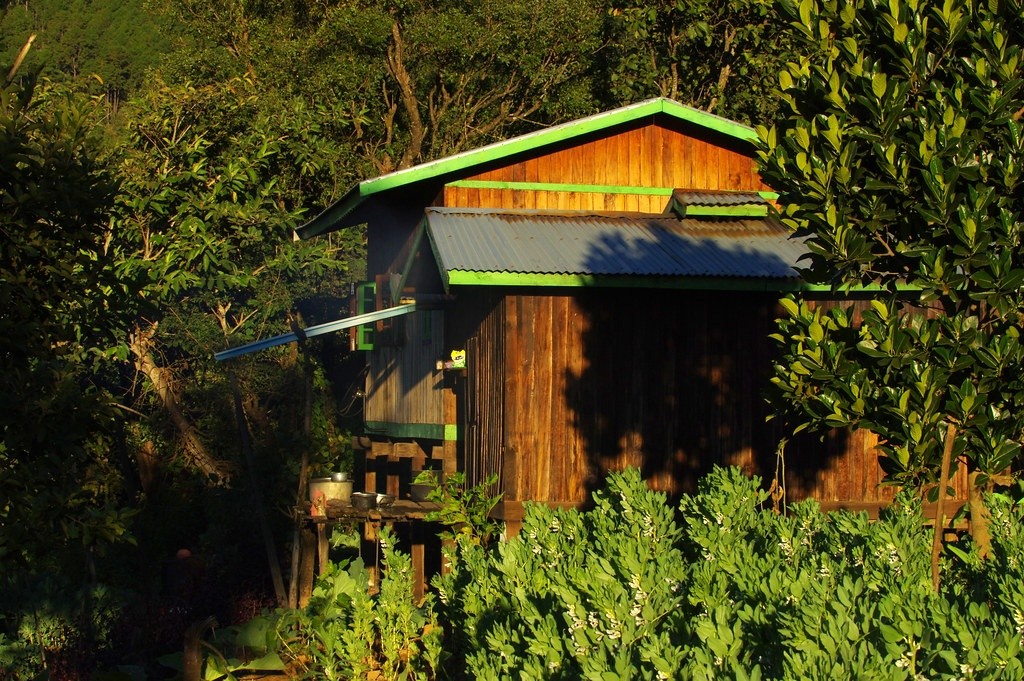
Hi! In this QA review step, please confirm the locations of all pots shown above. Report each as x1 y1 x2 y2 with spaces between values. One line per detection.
352 490 378 510
376 493 388 504
308 477 354 504
411 483 438 502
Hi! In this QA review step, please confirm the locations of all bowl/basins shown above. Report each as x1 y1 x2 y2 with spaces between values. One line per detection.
331 472 348 482
